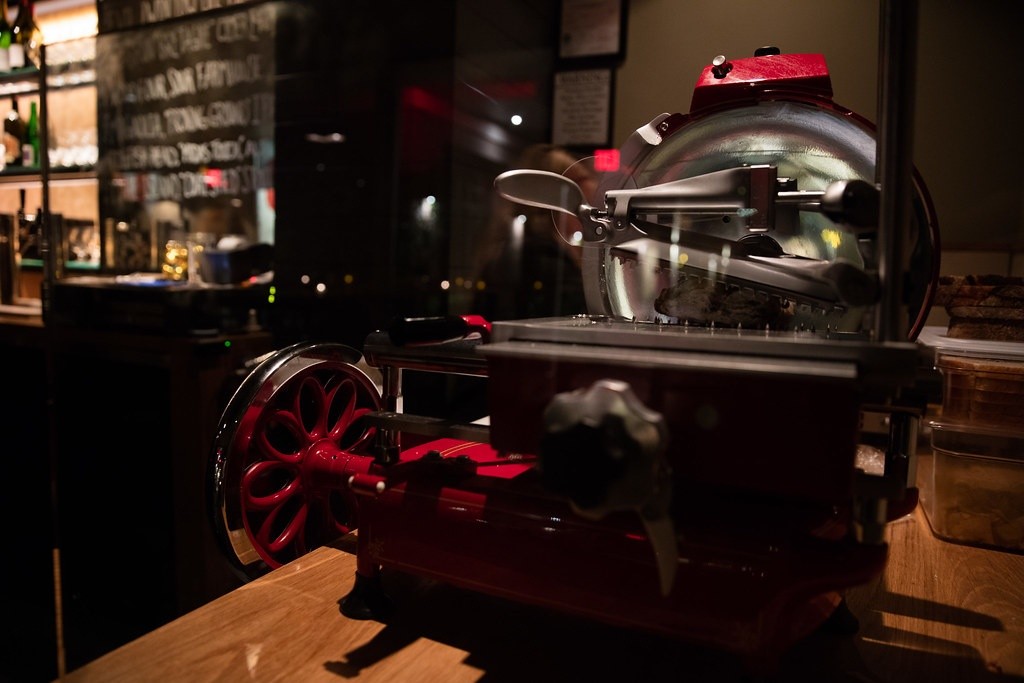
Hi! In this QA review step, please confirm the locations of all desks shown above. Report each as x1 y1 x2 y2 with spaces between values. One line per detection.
57 442 1024 683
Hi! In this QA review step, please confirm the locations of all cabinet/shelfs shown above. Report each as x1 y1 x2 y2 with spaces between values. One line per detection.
0 0 102 277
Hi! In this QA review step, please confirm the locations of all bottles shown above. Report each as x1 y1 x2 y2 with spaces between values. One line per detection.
3 96 40 168
0 0 42 74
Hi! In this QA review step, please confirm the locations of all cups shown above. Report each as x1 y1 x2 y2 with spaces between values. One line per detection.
186 232 216 282
